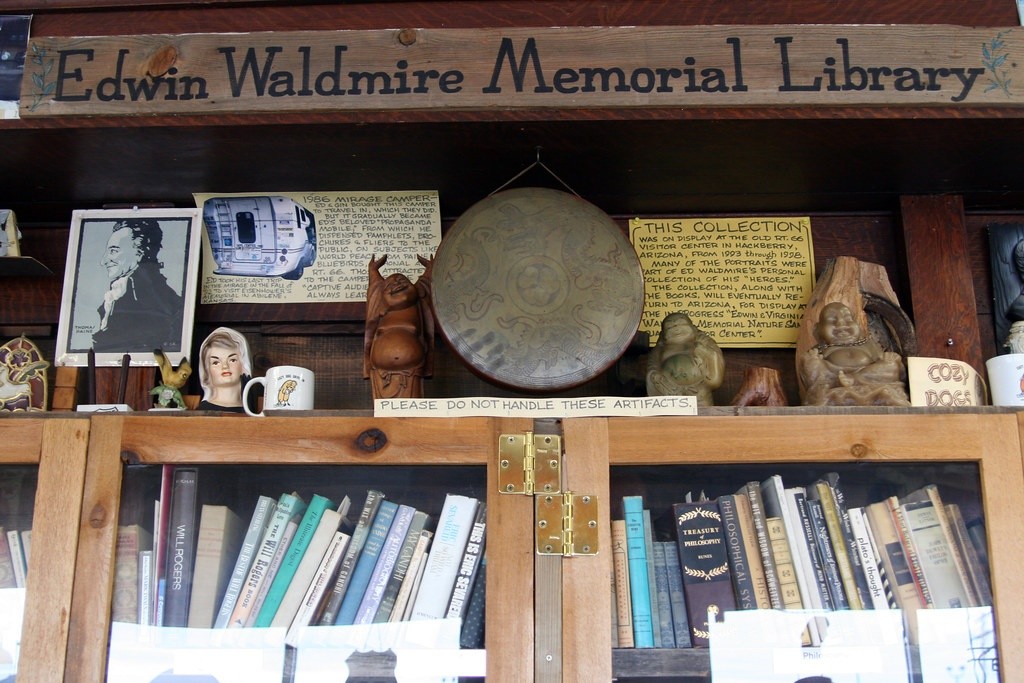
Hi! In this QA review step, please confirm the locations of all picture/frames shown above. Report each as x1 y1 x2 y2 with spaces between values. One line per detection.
55 208 205 368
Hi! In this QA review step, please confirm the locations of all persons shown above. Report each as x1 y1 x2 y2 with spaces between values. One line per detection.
366 254 435 398
646 313 724 407
199 326 257 413
801 301 909 406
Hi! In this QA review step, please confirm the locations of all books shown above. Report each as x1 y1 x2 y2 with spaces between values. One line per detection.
612 474 992 649
113 464 487 650
0 528 32 589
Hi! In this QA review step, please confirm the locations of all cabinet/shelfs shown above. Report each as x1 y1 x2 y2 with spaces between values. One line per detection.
0 406 1023 683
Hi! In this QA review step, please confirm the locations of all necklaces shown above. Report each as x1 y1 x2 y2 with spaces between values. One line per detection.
817 338 867 354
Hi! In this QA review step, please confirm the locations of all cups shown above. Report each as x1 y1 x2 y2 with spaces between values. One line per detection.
985 354 1024 406
243 366 314 417
